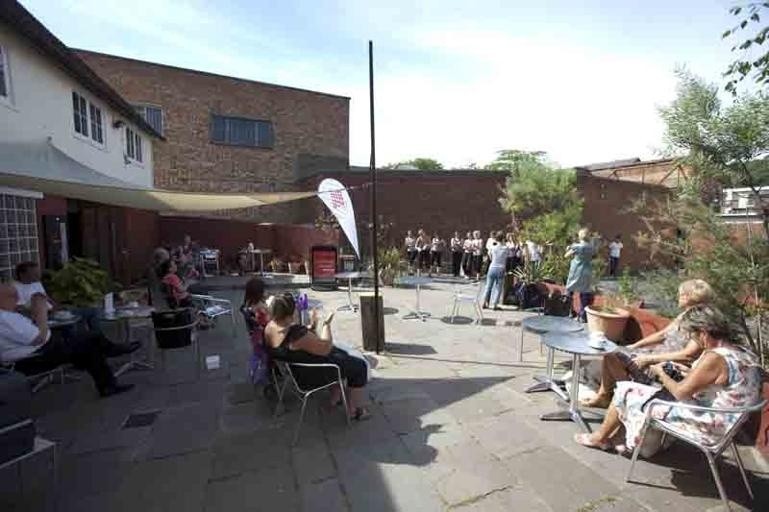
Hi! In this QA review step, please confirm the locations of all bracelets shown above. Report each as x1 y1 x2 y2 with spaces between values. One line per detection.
323 321 330 326
649 354 655 362
662 375 671 388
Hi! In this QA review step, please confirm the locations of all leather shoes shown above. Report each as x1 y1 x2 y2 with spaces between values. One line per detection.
99 385 134 397
123 341 141 354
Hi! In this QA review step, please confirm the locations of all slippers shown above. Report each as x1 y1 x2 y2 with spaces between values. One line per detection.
346 407 369 420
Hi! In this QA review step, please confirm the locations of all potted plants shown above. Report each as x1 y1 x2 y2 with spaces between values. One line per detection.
507 260 554 309
375 243 401 287
583 292 632 343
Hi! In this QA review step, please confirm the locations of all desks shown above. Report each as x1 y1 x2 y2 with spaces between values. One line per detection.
518 313 585 403
391 274 435 322
540 329 616 437
97 304 159 378
334 271 368 313
33 312 82 327
1 419 56 510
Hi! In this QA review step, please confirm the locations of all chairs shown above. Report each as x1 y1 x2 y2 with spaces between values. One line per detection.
150 246 270 362
247 326 284 402
1 359 70 393
625 393 769 512
448 282 485 325
270 358 352 447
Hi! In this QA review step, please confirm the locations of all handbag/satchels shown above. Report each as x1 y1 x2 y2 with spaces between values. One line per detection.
655 371 684 401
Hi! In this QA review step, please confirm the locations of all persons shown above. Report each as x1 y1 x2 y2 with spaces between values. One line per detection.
8 261 142 357
572 303 762 458
583 277 716 409
240 277 287 376
481 230 509 310
262 292 371 422
404 229 624 283
564 228 593 321
141 233 218 328
231 237 255 274
1 280 142 396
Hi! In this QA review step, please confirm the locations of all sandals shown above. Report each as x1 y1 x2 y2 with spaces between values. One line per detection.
581 398 610 408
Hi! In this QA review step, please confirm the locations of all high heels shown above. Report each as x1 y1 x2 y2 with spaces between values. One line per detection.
611 439 626 456
574 433 611 451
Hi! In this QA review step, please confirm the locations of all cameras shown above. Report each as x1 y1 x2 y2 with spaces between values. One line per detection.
654 362 683 383
